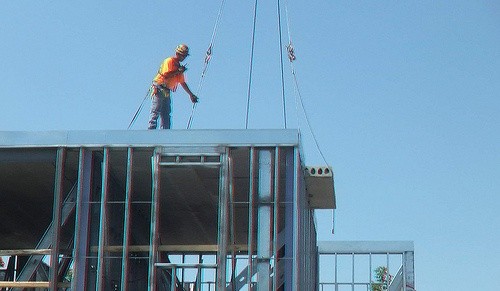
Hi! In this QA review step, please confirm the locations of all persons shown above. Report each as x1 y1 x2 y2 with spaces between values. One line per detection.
148 43 199 130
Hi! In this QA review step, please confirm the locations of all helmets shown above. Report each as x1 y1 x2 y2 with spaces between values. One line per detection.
175 44 188 57
